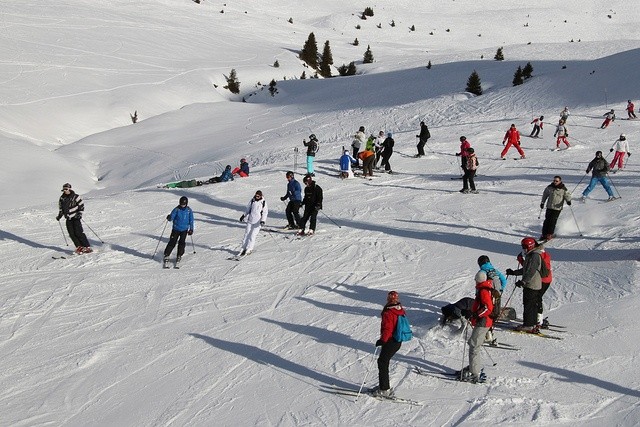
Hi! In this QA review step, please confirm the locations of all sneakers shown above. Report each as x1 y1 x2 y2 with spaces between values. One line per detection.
163 256 169 264
617 167 622 171
177 255 181 262
501 156 504 159
81 246 93 254
459 188 467 193
245 249 254 256
458 367 468 375
609 168 613 171
581 195 586 200
521 155 525 159
72 246 81 256
307 229 315 237
372 386 381 392
373 389 390 398
607 197 614 201
296 229 305 237
240 248 246 254
516 324 538 334
470 189 476 193
459 372 478 382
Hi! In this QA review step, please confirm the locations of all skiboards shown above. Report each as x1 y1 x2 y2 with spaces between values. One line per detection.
493 321 564 340
52 255 77 260
515 317 567 332
451 176 463 179
416 366 457 381
164 261 181 269
502 156 520 160
483 341 519 351
535 233 556 244
228 254 247 261
286 231 310 239
607 168 621 174
581 196 621 203
337 388 418 404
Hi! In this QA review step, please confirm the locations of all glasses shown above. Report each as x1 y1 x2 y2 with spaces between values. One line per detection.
287 176 291 178
255 195 260 198
180 203 186 206
304 183 308 185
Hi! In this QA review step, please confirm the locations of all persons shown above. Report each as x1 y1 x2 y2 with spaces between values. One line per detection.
356 150 375 177
500 123 527 163
369 290 407 397
581 151 615 204
556 104 573 130
162 191 194 271
478 255 506 342
529 116 545 138
232 158 250 178
303 133 320 177
459 147 479 193
609 132 632 172
295 173 324 237
598 109 616 129
415 121 430 157
364 130 377 153
338 150 357 179
56 183 93 255
162 178 203 189
624 99 638 121
281 170 304 231
351 124 366 164
209 165 235 184
455 135 471 177
237 189 267 262
374 130 385 170
552 120 572 152
540 175 572 241
440 273 492 383
380 131 394 173
440 296 475 337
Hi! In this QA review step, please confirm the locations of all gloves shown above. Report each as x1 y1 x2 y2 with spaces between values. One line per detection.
541 128 543 130
456 153 459 156
517 254 524 264
554 134 556 137
260 222 265 226
627 152 631 156
240 214 245 222
566 134 568 137
56 214 62 221
375 340 384 347
515 280 523 288
518 140 521 145
167 215 171 221
503 141 505 144
610 148 614 152
77 214 82 219
297 202 302 207
506 269 514 275
281 197 285 201
187 230 193 235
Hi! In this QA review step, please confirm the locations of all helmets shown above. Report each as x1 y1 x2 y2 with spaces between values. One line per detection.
286 171 294 181
511 124 515 127
303 175 312 186
521 238 536 252
387 291 399 302
559 120 564 125
478 255 490 267
226 165 231 169
309 134 315 140
180 196 188 208
565 106 569 110
467 147 474 154
240 158 246 162
459 135 466 142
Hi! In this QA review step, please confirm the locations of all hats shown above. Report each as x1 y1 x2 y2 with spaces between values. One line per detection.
61 182 72 192
475 271 487 283
256 190 262 196
344 149 349 156
619 132 626 137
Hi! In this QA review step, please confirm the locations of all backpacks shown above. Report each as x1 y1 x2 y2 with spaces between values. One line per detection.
530 252 549 278
394 314 413 343
478 286 501 319
